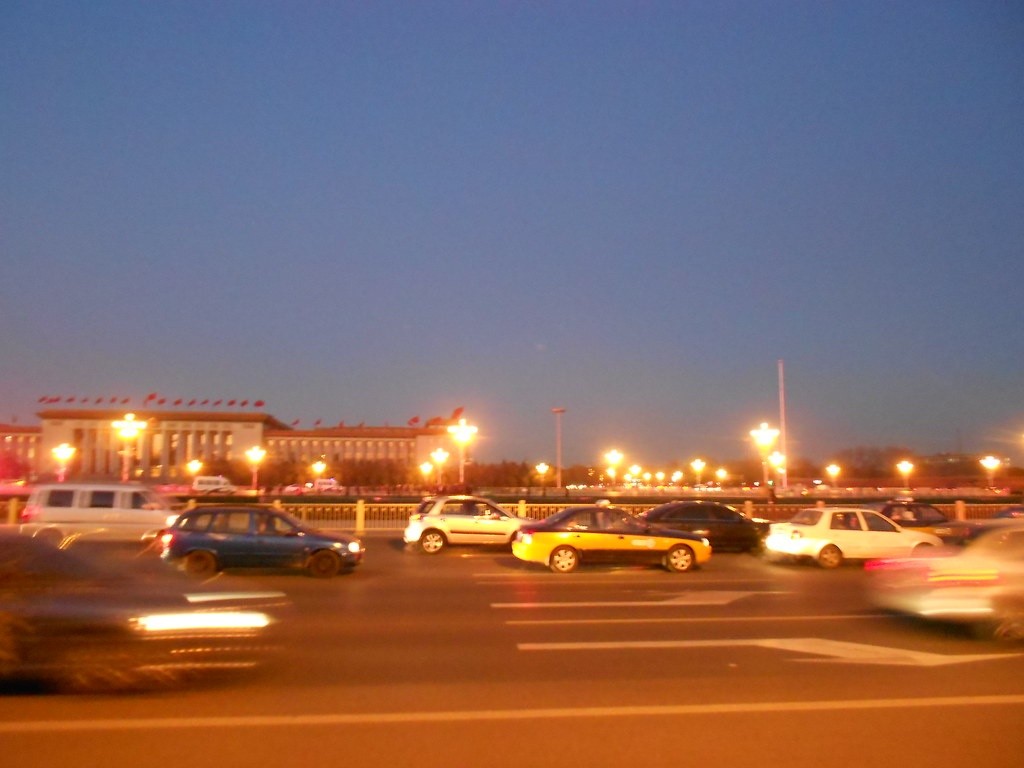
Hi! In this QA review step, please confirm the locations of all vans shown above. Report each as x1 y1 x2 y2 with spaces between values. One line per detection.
17 483 180 549
192 475 236 495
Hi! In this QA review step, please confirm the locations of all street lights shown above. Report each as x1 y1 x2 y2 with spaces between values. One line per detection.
51 444 77 480
416 418 479 486
604 418 998 493
111 414 150 482
246 445 267 491
551 407 567 487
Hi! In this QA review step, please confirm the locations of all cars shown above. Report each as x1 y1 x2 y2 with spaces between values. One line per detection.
865 529 1024 643
766 508 943 570
513 503 714 571
850 500 972 543
284 478 346 494
0 531 276 694
620 502 774 552
404 496 540 555
156 505 364 580
